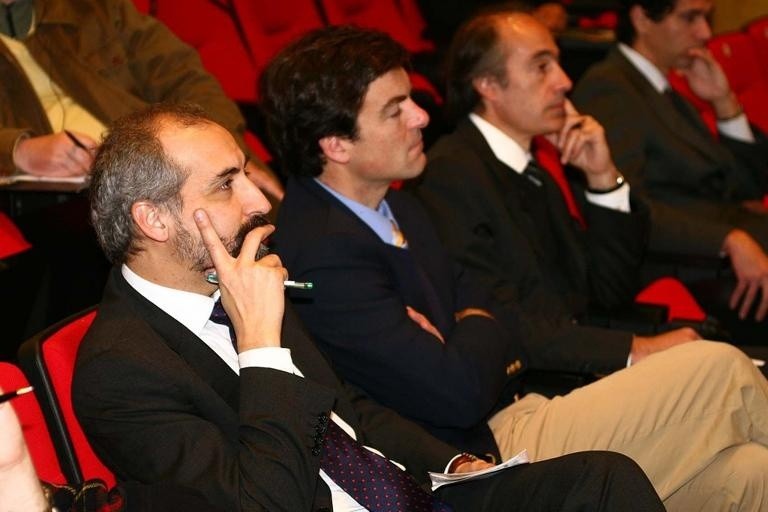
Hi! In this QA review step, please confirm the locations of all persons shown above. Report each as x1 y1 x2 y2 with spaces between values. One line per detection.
393 11 767 398
0 390 103 512
570 0 768 321
71 101 669 511
1 0 283 247
258 24 768 512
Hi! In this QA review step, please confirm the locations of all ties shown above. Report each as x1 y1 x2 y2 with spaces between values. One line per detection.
389 219 411 254
209 295 447 508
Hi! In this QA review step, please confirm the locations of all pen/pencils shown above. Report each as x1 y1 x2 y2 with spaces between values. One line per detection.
207 274 313 289
0 386 33 403
63 129 88 151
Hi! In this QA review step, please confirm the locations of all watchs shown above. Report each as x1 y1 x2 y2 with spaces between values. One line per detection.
447 454 479 474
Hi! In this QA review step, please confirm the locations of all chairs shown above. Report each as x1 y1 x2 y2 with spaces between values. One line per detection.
534 133 723 340
321 0 437 54
745 14 768 62
151 0 274 166
0 212 33 272
230 0 326 74
0 358 77 512
390 72 455 191
18 302 125 512
704 32 768 136
668 68 768 215
